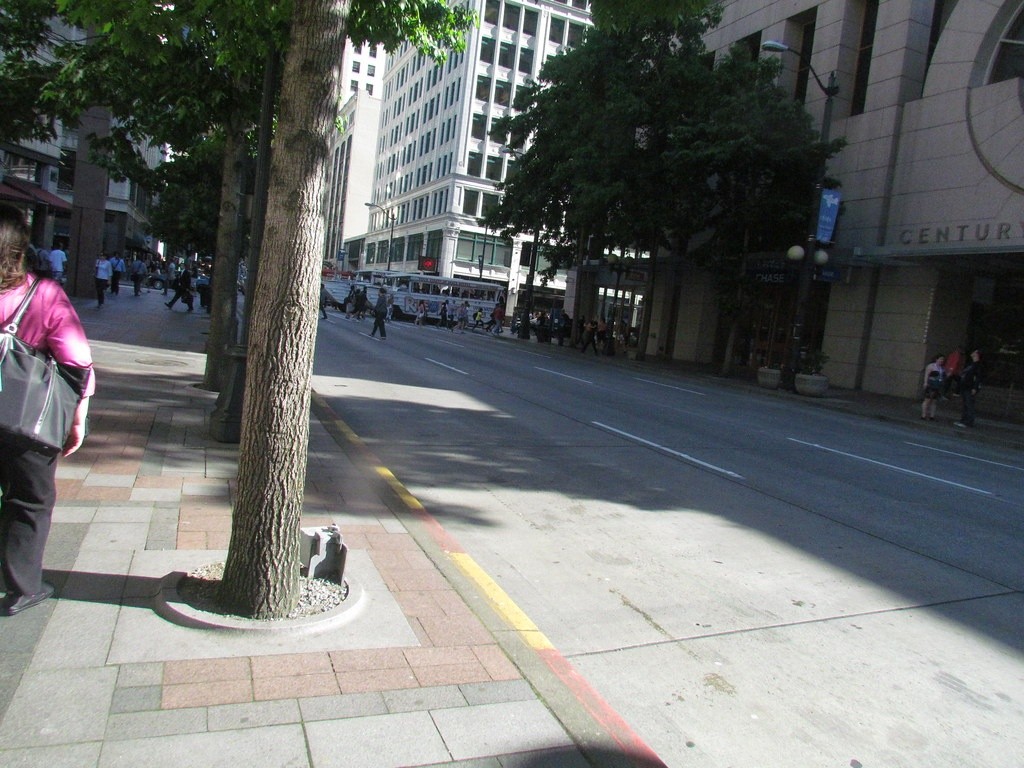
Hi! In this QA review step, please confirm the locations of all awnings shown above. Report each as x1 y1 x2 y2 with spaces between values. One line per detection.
126 238 158 256
0 176 72 210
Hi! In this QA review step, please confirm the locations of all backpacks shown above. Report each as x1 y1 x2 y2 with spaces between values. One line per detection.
966 374 984 405
927 370 943 391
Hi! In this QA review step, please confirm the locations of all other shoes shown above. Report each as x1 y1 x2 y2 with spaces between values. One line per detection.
2 580 55 614
164 303 172 308
187 308 193 311
161 292 166 295
921 415 939 422
953 420 967 428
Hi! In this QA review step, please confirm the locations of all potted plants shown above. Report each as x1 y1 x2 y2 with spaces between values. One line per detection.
795 348 832 397
757 355 781 388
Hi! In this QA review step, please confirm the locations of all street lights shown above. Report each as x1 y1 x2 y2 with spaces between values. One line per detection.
762 39 837 361
502 149 539 338
365 203 396 272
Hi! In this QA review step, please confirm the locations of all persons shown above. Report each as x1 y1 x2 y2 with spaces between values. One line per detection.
320 283 329 320
415 283 630 357
344 284 394 341
27 240 213 313
0 202 96 613
920 345 987 428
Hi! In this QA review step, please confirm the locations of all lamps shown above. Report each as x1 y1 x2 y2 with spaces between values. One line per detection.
761 41 844 97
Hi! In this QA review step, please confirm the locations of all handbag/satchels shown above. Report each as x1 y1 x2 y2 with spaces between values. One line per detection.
473 313 478 320
90 258 101 278
130 272 134 281
376 303 388 319
1 273 93 456
181 291 194 303
418 312 425 318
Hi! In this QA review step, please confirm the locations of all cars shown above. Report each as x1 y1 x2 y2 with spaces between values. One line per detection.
146 271 209 294
